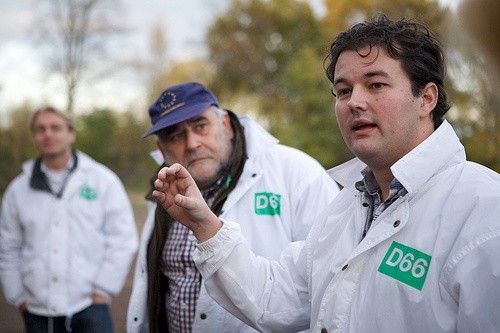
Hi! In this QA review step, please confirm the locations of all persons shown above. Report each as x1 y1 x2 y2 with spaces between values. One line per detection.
152 14 500 332
1 106 139 332
125 82 340 333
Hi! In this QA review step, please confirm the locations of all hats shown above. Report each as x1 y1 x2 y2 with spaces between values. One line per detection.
142 82 219 141
